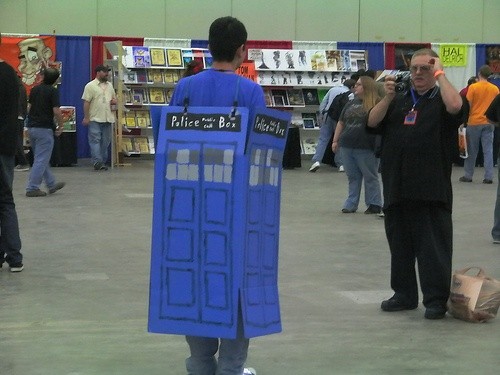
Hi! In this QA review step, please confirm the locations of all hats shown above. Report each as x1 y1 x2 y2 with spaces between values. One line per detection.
95 65 111 72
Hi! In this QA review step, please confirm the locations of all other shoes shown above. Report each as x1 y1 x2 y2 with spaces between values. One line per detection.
460 176 472 182
492 239 500 244
49 182 65 194
26 189 46 197
338 165 345 172
483 179 493 184
94 164 108 171
364 204 381 215
341 208 356 213
243 367 256 375
308 161 320 172
381 295 418 311
425 307 446 319
9 264 24 272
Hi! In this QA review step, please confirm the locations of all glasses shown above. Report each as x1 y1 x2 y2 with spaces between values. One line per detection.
354 83 362 86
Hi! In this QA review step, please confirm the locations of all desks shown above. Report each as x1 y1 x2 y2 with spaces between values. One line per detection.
28 131 78 167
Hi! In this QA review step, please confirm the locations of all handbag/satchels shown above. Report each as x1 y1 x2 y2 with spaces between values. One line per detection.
448 265 500 323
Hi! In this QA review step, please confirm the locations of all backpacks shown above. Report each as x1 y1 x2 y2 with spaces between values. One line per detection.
322 91 353 121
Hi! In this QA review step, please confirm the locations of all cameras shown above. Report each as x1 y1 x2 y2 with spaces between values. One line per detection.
393 74 411 93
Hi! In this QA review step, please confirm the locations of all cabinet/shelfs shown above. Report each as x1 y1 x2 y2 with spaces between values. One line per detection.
104 41 369 167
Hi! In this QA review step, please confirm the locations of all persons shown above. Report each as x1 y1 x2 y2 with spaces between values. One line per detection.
365 49 470 320
332 76 386 214
309 79 356 172
81 66 117 171
484 93 500 244
183 60 203 77
461 64 499 184
459 76 479 96
0 58 27 272
26 68 66 197
169 16 266 375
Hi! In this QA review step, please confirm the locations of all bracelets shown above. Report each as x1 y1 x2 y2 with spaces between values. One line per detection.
434 70 445 80
